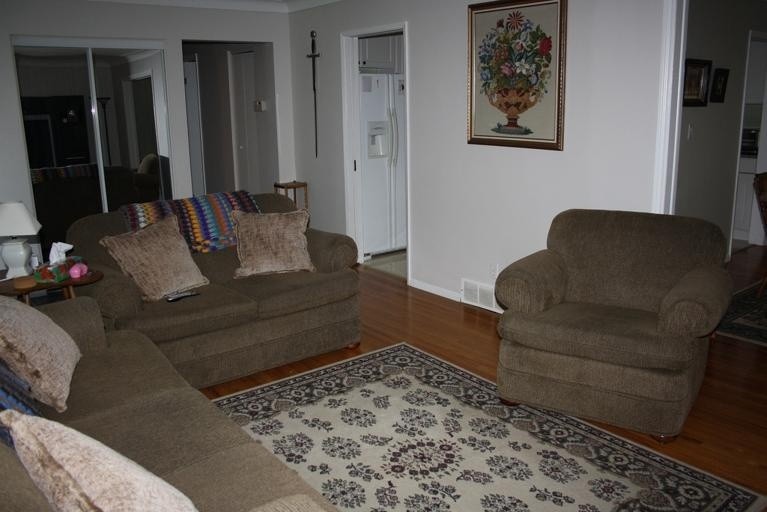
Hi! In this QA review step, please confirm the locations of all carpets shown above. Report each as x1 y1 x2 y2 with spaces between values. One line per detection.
211 340 767 512
713 278 767 347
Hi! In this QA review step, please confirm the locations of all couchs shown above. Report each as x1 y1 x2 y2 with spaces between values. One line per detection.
33 150 171 263
0 295 341 512
64 193 364 391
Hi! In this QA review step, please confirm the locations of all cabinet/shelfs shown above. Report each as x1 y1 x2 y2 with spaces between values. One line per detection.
357 33 405 75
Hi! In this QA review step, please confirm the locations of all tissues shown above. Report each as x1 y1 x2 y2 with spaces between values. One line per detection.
34 241 85 284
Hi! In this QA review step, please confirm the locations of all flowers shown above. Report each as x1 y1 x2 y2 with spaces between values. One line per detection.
478 10 553 102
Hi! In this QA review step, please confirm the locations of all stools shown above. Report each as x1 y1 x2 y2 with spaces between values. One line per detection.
274 179 310 229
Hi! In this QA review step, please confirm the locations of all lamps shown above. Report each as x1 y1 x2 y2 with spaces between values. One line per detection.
0 201 43 280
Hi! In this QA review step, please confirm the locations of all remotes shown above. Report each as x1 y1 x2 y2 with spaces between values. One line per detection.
165 289 202 302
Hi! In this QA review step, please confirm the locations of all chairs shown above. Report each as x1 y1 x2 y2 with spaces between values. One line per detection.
495 208 735 444
752 172 767 299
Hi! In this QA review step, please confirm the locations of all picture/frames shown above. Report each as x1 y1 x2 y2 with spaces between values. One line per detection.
709 67 731 104
466 0 568 149
682 58 713 107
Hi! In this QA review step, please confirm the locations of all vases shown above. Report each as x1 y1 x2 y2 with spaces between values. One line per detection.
487 87 542 135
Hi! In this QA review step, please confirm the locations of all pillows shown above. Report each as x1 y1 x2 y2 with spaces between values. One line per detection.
0 408 199 512
0 294 85 414
231 206 317 281
98 214 210 303
